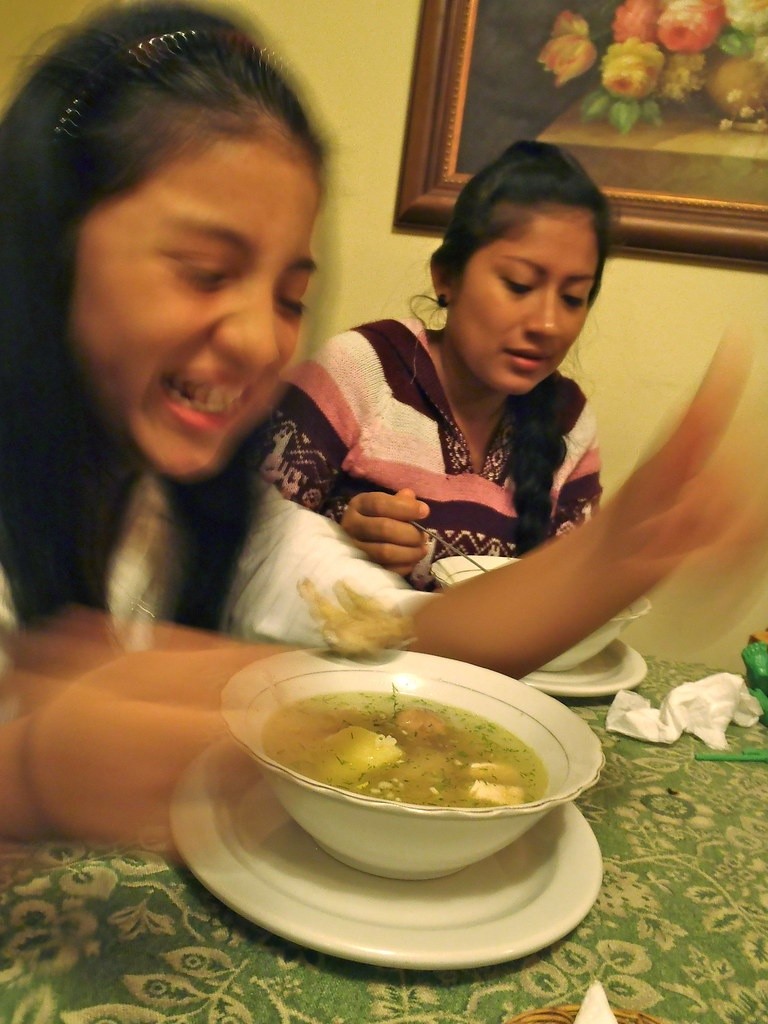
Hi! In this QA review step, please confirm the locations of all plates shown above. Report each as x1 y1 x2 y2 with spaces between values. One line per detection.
514 637 647 697
171 734 603 971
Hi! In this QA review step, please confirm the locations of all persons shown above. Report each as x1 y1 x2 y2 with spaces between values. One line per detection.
257 139 612 609
1 4 755 871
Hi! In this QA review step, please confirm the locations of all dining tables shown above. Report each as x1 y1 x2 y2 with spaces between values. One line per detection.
2 649 768 1024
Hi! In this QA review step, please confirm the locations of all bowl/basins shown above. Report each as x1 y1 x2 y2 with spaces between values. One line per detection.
429 555 651 674
217 647 609 889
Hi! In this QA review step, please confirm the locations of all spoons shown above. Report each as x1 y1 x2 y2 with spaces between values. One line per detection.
130 597 415 664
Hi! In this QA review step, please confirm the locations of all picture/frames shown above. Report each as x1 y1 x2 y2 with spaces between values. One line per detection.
391 1 767 268
